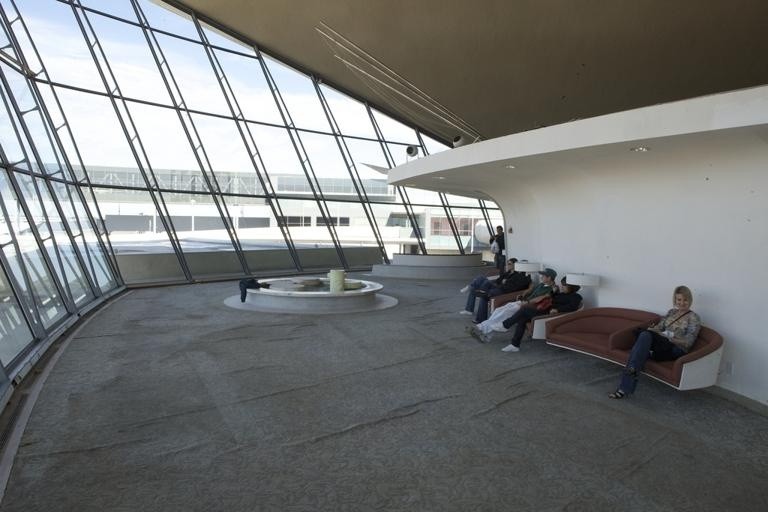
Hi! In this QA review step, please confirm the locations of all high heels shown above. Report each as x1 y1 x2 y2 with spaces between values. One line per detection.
607 389 631 399
621 366 640 379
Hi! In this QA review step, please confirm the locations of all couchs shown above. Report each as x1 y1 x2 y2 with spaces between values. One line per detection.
472 274 723 393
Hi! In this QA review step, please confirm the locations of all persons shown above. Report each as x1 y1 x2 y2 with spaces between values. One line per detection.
457 257 516 314
462 268 559 344
492 276 582 353
471 271 530 323
607 285 701 399
490 225 507 274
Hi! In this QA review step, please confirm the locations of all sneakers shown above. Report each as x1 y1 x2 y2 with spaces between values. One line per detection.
490 321 509 332
501 344 520 352
460 285 473 294
460 309 491 344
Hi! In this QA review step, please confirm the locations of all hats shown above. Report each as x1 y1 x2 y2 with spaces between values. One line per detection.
538 268 557 278
